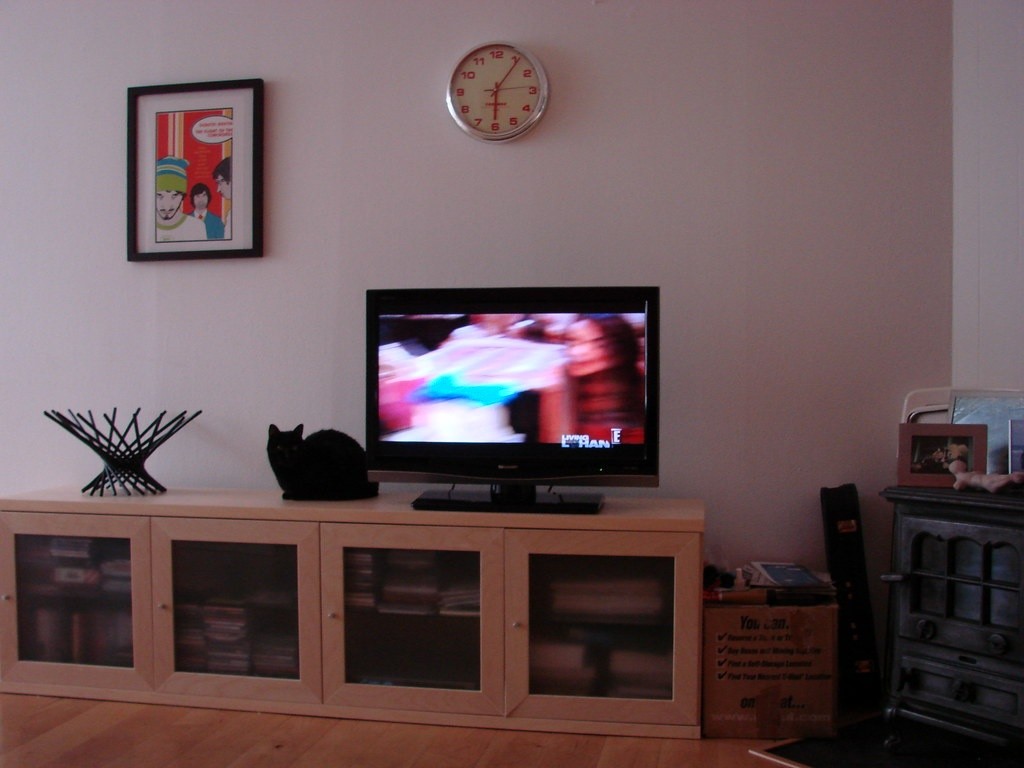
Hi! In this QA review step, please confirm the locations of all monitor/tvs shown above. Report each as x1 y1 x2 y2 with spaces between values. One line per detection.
366 285 662 515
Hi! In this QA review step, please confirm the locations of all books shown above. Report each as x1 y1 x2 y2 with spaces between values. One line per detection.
176 602 298 676
743 561 837 595
345 551 480 617
20 537 132 659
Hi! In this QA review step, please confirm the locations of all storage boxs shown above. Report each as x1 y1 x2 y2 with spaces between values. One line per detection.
878 486 1024 756
703 594 838 738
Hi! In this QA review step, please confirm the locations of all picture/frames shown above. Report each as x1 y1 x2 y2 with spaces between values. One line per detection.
896 423 988 485
125 78 263 260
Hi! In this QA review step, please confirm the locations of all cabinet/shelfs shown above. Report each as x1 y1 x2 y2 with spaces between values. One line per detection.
0 485 704 740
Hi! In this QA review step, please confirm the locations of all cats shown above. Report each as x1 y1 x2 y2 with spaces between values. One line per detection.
266 424 379 501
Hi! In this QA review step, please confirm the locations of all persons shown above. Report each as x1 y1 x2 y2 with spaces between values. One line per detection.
911 444 968 473
380 312 645 444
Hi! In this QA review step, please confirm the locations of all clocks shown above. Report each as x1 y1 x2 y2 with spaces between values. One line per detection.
445 39 550 142
